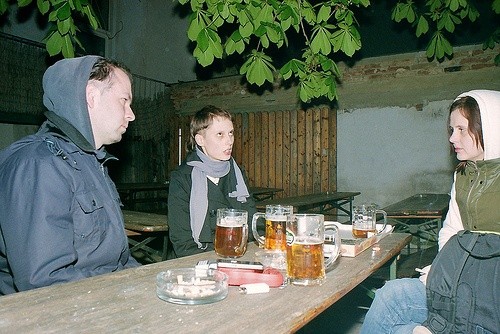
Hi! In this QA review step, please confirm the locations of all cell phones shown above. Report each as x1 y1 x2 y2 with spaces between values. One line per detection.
217 258 263 270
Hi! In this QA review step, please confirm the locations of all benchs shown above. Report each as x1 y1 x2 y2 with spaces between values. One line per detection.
343 212 384 227
124 228 158 263
389 218 412 255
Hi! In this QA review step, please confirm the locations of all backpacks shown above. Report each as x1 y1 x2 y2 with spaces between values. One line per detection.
422 228 500 334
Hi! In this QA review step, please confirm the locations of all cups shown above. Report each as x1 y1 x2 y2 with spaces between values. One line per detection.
286 214 342 287
214 208 248 259
251 205 293 253
352 205 387 238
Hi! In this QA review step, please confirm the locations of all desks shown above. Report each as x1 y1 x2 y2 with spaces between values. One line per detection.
0 233 413 334
121 210 169 263
252 186 285 201
377 192 451 253
256 190 361 221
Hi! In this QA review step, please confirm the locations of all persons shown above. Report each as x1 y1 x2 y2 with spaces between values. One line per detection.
0 54 144 296
169 105 266 259
360 89 500 334
